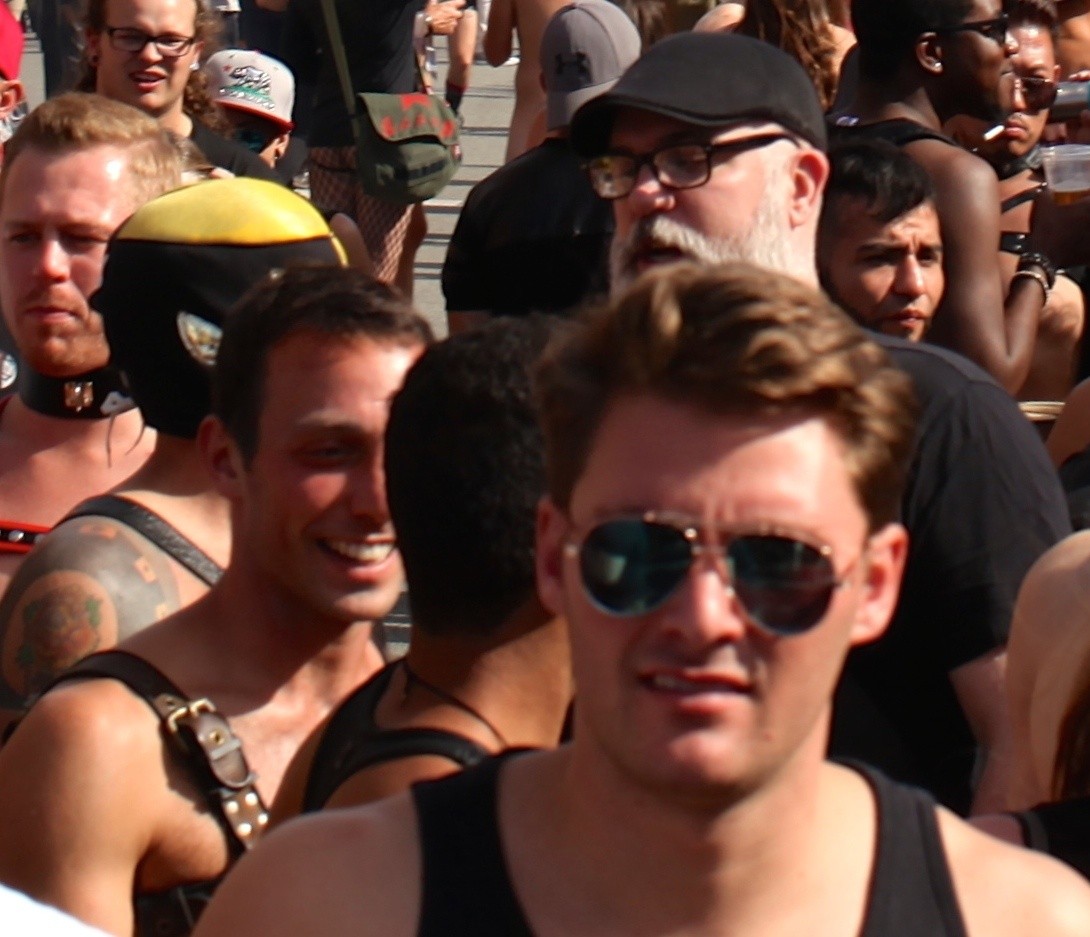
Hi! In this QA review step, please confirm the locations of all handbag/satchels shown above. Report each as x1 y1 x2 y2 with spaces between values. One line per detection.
348 92 462 205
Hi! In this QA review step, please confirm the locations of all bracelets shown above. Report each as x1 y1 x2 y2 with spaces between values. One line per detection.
1009 270 1049 309
1013 251 1056 289
422 10 433 38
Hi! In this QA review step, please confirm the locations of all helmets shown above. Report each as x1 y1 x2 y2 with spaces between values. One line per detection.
103 178 348 438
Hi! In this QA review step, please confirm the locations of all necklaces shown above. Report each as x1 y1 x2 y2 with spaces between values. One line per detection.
398 659 514 755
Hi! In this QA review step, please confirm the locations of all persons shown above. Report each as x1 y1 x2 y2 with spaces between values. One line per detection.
0 0 1090 937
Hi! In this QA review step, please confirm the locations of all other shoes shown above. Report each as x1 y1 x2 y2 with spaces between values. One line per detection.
423 46 441 77
474 51 521 66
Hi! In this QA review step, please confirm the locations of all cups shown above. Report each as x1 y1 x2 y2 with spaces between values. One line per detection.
1038 144 1090 205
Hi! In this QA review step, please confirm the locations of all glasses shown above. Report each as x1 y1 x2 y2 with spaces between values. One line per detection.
1013 76 1065 113
579 130 801 199
214 130 283 155
97 20 197 57
558 511 866 638
933 10 1010 45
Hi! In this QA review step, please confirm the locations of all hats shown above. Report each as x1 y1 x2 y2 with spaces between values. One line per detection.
0 1 24 81
203 49 295 131
567 32 826 156
539 0 641 131
